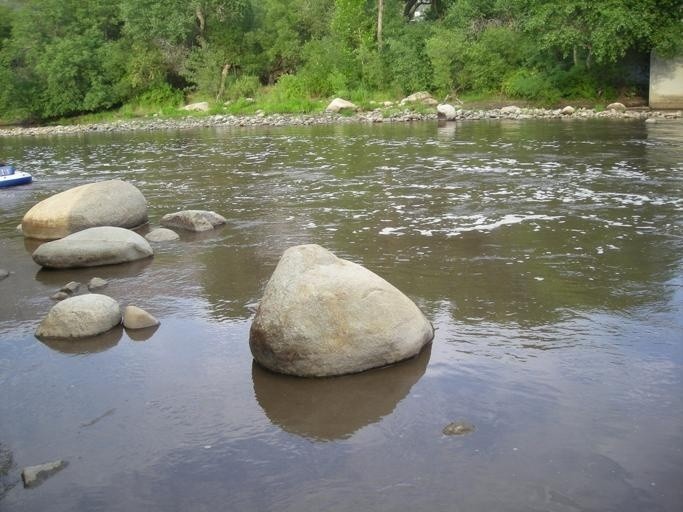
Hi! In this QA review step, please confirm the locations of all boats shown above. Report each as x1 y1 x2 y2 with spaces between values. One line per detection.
0 164 33 187
